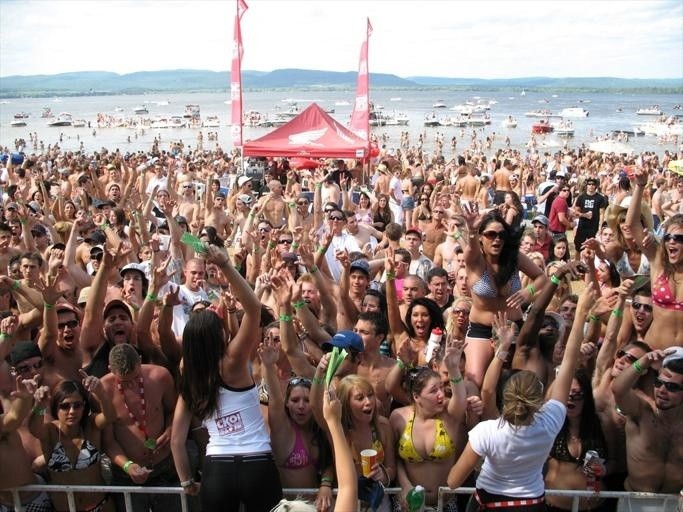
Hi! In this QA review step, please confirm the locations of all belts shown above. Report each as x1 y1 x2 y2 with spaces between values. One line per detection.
204 453 277 462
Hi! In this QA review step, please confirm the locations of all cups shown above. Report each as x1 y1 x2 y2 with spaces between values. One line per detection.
360 449 377 477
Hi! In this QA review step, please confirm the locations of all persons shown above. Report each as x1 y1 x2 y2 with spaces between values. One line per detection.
0 98 682 511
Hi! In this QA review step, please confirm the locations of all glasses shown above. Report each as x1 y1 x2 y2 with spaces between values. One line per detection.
0 178 683 412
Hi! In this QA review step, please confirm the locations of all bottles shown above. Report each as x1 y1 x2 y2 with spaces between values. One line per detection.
406 485 425 511
425 327 442 363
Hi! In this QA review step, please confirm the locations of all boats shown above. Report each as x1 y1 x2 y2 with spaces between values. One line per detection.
502 102 681 137
